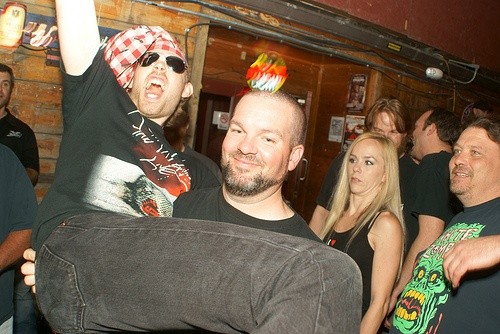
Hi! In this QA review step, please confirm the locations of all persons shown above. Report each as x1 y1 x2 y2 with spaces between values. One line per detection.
0 143 37 334
0 63 40 187
308 94 500 334
21 89 323 293
30 1 362 334
163 103 222 187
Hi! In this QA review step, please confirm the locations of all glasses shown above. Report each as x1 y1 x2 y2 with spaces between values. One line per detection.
139 51 188 74
